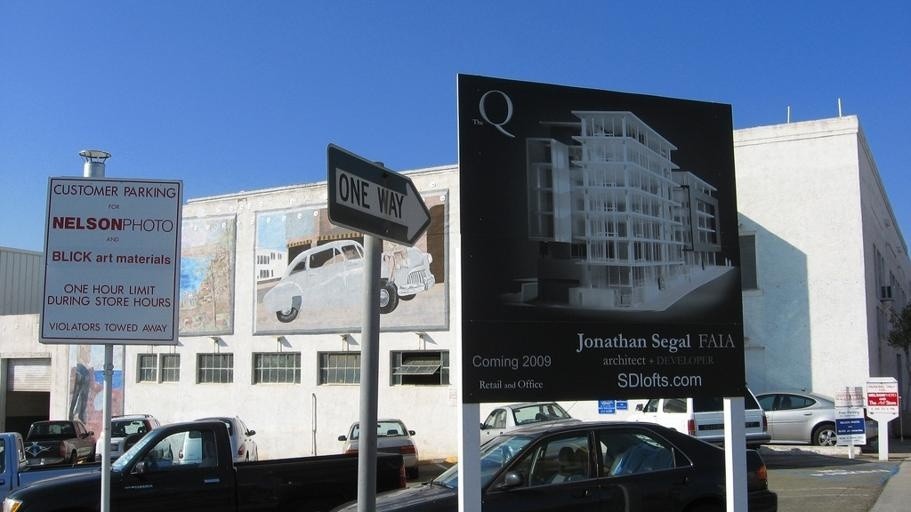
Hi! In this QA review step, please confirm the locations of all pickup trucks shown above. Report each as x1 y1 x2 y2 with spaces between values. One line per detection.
1 419 406 511
0 420 96 512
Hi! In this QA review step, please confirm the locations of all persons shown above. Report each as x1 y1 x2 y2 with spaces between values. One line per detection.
69 345 92 425
381 240 398 288
394 244 411 270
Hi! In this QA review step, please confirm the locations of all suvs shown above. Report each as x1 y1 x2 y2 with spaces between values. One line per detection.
93 413 174 464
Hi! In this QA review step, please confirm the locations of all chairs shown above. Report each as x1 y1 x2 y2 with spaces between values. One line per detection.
548 446 588 483
198 440 217 466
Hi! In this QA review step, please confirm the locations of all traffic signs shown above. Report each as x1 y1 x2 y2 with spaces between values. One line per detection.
325 141 433 249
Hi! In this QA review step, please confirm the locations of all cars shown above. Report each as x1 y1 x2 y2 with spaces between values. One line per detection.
178 415 259 466
336 418 420 480
332 418 779 512
